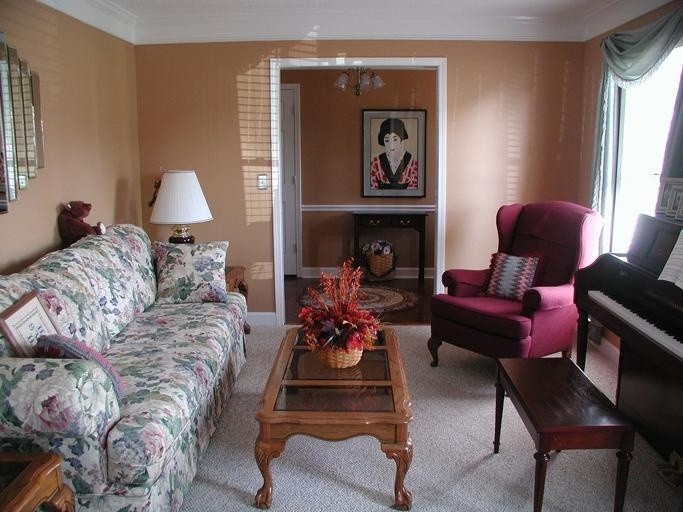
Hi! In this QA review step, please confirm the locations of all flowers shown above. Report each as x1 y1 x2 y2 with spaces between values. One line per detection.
359 239 399 270
296 255 383 352
289 380 377 419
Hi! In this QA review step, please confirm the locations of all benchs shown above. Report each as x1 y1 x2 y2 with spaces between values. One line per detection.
491 355 636 512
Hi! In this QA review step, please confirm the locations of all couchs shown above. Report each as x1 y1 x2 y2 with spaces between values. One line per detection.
0 222 248 512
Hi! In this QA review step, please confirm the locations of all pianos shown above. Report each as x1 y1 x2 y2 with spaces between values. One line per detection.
576 212 683 469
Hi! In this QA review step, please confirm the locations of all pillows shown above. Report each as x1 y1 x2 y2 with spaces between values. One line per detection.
151 239 230 305
32 333 124 402
479 250 546 304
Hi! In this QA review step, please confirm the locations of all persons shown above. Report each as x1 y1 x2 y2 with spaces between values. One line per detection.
371 118 417 190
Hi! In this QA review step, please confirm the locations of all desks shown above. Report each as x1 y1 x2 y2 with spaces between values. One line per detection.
225 265 251 334
351 211 429 286
0 451 77 512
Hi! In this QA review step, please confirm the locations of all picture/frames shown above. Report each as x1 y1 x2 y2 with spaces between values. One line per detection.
361 108 426 199
654 176 682 222
0 288 62 358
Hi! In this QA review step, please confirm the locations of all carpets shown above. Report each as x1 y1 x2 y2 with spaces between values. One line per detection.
298 280 419 315
174 324 681 511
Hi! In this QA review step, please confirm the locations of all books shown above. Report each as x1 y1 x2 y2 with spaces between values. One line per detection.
658 228 683 291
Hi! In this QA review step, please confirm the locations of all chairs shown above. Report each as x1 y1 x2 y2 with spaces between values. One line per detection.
426 201 603 396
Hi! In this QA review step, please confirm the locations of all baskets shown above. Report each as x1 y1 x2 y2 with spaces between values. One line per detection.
316 347 363 369
367 254 393 278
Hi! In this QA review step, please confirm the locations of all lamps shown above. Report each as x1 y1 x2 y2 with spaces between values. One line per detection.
147 168 214 243
332 69 386 96
